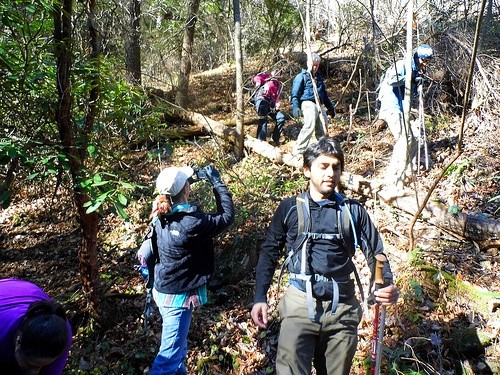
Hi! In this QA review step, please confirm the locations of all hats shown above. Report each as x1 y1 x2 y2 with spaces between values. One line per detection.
156 166 194 195
416 43 434 58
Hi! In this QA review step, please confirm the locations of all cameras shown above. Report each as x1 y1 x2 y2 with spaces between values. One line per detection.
196 170 208 179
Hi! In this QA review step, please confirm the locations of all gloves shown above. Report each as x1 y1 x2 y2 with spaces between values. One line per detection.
326 108 336 117
293 107 302 119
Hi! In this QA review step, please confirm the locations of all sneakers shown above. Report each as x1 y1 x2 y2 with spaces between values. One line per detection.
273 133 286 141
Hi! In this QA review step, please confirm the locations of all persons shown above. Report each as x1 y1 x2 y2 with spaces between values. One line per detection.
251 140 398 373
292 53 335 155
254 72 285 145
377 44 433 186
0 278 72 374
147 166 235 374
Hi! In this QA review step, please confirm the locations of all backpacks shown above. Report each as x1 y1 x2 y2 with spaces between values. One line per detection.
137 237 156 314
